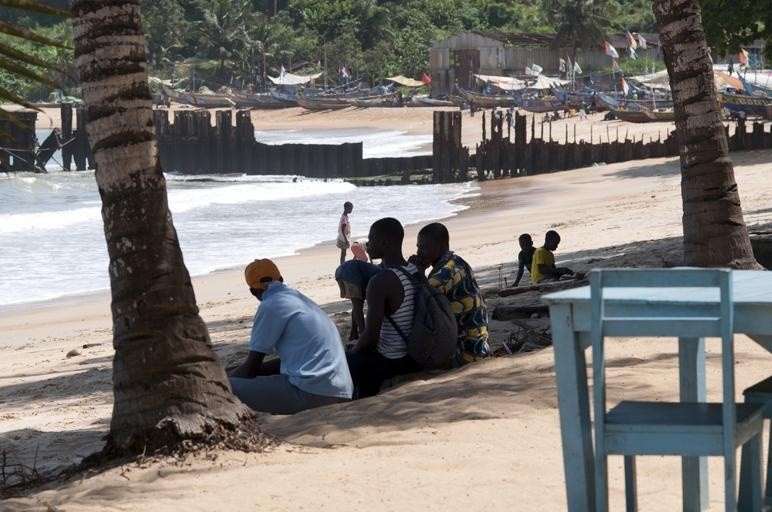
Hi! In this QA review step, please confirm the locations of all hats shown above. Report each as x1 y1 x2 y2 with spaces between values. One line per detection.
243 258 282 290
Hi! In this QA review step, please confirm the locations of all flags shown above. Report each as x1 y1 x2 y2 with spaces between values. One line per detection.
423 72 430 84
340 66 351 79
727 48 760 76
524 30 648 98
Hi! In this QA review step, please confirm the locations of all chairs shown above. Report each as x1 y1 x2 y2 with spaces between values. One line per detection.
739 374 772 511
585 268 764 510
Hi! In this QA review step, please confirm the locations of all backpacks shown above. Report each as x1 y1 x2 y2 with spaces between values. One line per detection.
385 262 458 368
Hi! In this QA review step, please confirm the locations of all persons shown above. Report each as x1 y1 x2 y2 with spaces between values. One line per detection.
511 233 536 287
350 241 373 264
408 222 491 364
377 85 600 129
226 258 355 416
346 217 426 399
335 260 382 341
530 230 573 285
335 201 353 264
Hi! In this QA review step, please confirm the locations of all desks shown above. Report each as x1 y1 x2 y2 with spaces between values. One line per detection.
540 271 772 510
